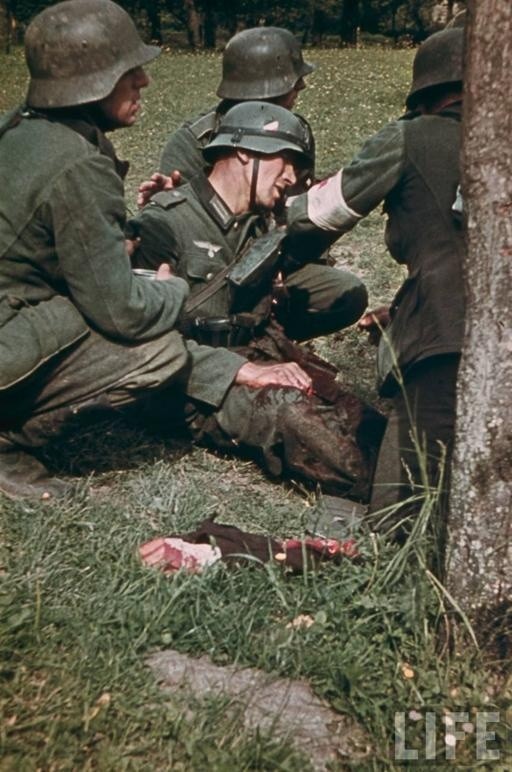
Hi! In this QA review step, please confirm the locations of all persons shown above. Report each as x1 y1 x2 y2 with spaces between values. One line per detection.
0 0 190 504
137 27 369 343
123 99 389 502
134 522 369 572
286 24 465 543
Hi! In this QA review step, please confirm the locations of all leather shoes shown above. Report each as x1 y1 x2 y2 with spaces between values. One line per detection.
0 445 88 514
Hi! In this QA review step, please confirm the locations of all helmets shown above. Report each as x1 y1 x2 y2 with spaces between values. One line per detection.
216 26 315 101
23 0 162 110
404 27 465 111
201 100 316 166
